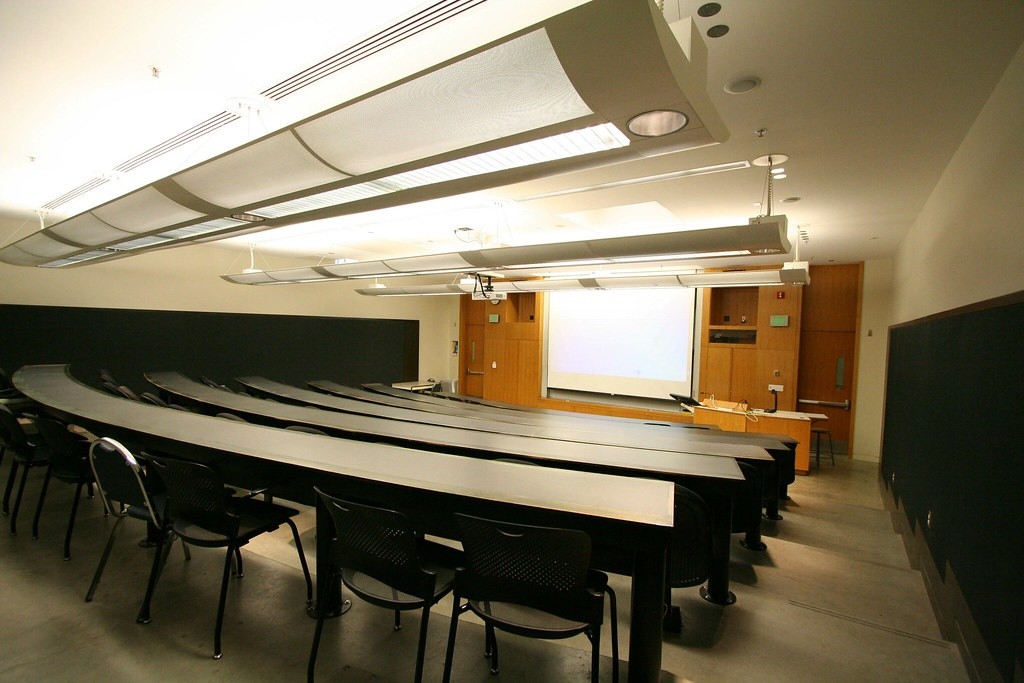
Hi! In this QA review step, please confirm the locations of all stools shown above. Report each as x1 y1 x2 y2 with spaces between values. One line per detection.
811 428 835 467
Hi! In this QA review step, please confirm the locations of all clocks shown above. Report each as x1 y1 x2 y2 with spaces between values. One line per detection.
490 299 500 305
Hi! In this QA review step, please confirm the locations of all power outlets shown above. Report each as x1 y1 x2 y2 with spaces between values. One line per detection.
773 370 779 375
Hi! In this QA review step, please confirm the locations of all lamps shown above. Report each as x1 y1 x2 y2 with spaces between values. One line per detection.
0 0 813 299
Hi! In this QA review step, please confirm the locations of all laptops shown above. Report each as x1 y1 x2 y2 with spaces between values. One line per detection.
670 393 702 407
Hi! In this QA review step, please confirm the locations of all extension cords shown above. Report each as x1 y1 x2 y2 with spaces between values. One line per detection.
718 407 733 412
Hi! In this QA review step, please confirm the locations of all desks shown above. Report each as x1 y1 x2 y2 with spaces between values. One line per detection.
11 365 830 683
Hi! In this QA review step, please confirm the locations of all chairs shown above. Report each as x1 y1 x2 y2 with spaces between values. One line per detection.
0 403 94 537
84 438 237 624
31 414 110 561
305 486 492 683
662 482 715 634
443 511 619 683
420 383 442 397
135 449 315 660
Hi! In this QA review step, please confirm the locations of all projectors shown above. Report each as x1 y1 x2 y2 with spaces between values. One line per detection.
472 291 507 300
